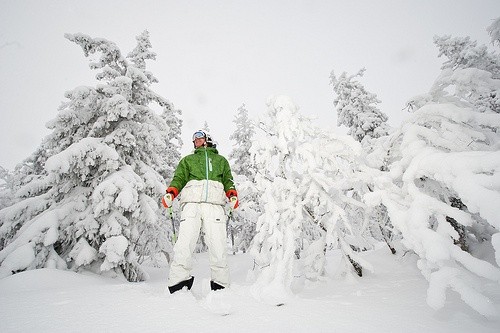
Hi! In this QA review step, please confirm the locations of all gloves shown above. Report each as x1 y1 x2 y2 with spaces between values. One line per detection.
226 190 240 209
162 187 179 208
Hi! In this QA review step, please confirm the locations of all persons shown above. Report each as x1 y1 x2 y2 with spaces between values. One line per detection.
161 129 239 294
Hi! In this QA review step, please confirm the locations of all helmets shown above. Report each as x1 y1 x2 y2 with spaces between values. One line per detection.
192 129 213 149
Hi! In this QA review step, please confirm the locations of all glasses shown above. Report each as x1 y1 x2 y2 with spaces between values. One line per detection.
192 132 205 141
